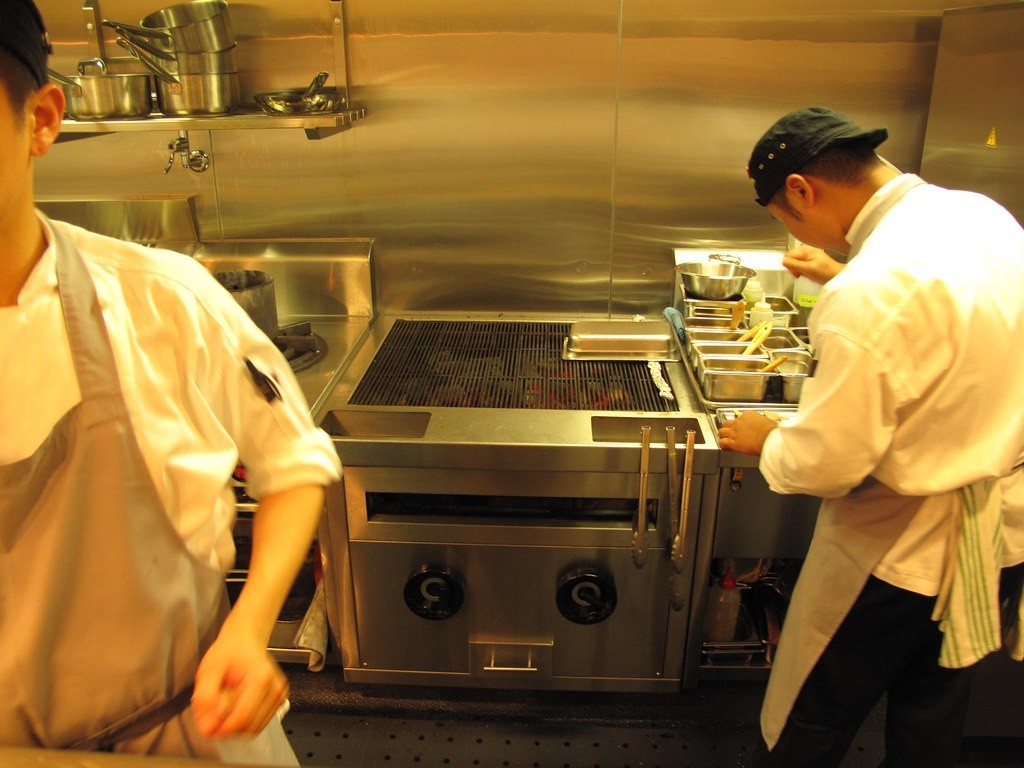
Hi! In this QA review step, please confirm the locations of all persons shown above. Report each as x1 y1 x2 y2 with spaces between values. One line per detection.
718 106 1024 768
0 0 343 768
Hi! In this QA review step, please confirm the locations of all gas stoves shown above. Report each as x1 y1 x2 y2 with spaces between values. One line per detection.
191 238 381 425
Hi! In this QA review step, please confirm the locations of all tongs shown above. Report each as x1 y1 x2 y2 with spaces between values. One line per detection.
631 424 697 576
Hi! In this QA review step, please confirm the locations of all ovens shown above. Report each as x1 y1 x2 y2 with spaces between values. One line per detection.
328 464 703 711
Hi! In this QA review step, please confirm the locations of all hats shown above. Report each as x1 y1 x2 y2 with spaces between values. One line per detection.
0 0 53 88
746 106 888 207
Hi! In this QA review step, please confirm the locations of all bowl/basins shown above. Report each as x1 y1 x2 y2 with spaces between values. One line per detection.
676 261 757 301
682 295 814 408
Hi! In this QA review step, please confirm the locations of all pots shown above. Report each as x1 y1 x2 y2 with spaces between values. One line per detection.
252 70 346 116
46 0 243 118
210 269 280 343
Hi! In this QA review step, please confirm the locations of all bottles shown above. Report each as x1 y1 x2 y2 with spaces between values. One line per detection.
703 566 741 642
